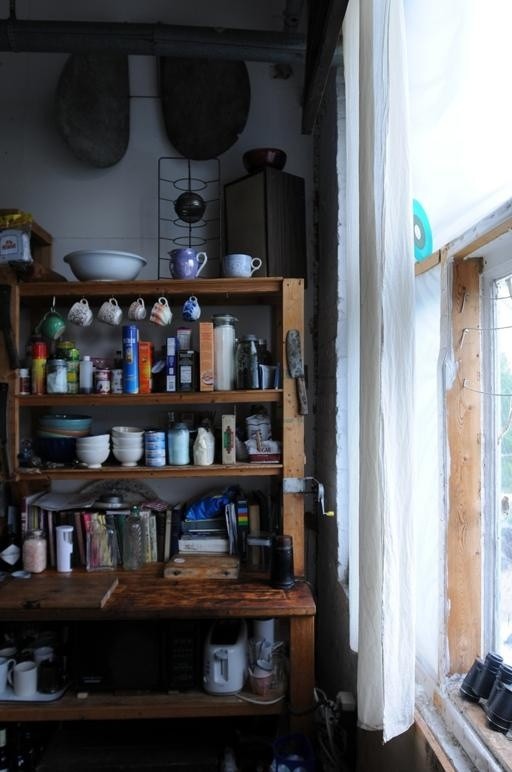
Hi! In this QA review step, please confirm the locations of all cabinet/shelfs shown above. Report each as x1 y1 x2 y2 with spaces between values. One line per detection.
0 276 306 577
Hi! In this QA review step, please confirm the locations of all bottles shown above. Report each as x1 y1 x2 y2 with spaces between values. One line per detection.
211 312 274 390
221 749 238 772
268 535 297 590
169 423 191 466
15 325 142 396
2 505 146 574
0 721 38 772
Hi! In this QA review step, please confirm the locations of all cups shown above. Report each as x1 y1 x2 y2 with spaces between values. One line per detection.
65 299 96 327
43 311 65 343
142 431 167 466
128 298 147 322
183 297 202 323
149 298 174 328
98 295 124 326
0 647 62 695
168 249 208 281
247 616 277 694
221 253 264 279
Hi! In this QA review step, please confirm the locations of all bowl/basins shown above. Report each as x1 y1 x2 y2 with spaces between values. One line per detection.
112 426 145 466
60 249 147 281
37 414 93 438
75 433 110 468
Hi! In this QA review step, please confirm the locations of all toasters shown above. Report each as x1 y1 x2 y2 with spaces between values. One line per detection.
199 618 248 693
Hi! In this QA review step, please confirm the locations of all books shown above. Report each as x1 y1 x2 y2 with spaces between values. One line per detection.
20 490 278 581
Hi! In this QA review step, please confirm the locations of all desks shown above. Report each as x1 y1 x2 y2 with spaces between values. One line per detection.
0 560 318 772
